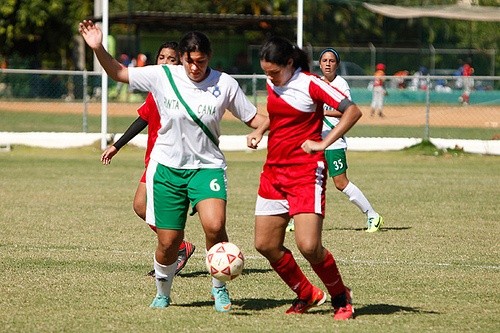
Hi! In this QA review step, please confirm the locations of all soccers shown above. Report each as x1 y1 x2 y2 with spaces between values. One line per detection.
206 242 244 282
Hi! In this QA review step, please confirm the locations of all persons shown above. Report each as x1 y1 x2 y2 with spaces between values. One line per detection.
280 49 383 234
100 41 196 279
78 18 269 313
121 47 475 121
246 39 363 319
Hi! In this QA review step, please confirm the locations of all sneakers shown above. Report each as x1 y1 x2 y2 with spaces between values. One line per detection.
286 286 327 313
150 294 172 307
331 286 355 320
174 240 196 275
284 219 295 231
211 283 231 311
365 213 383 232
